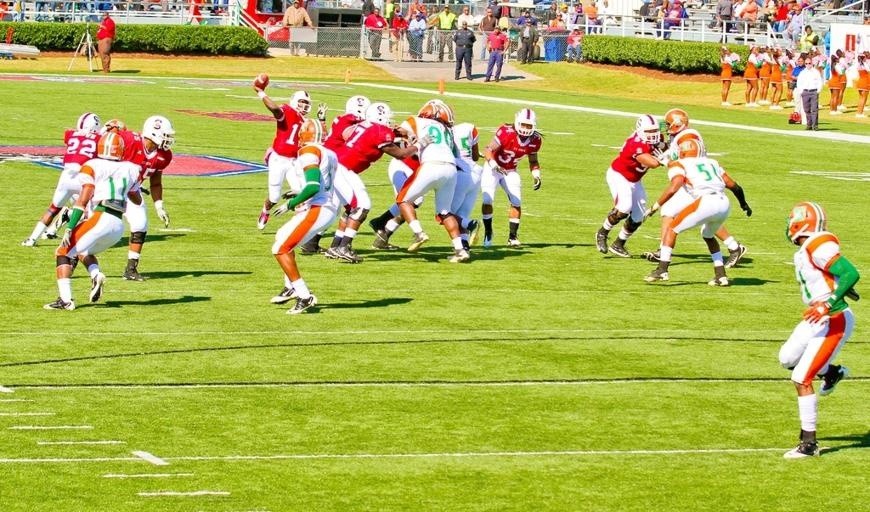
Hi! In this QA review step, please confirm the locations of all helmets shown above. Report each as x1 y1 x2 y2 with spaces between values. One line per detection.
514 108 536 136
345 95 393 126
79 112 175 159
289 91 312 115
419 99 456 124
785 201 826 245
297 118 325 147
638 109 707 154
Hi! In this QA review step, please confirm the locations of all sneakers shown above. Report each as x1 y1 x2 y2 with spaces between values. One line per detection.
720 100 864 120
44 298 76 312
304 221 522 261
88 272 106 303
818 364 848 396
122 267 144 282
594 234 745 285
257 208 270 230
270 288 319 312
783 442 819 459
24 210 66 246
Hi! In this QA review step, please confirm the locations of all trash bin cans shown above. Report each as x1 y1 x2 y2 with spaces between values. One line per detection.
542 29 570 62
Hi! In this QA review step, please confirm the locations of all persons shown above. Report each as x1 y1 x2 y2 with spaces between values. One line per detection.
96 12 116 73
268 119 339 315
361 0 869 131
641 108 749 268
21 111 174 312
642 133 754 290
778 200 862 463
254 73 544 265
594 114 672 259
283 0 315 57
0 0 283 25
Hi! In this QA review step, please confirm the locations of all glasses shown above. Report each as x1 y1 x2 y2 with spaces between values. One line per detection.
805 62 811 65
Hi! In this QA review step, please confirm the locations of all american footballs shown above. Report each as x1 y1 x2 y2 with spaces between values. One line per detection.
255 74 269 91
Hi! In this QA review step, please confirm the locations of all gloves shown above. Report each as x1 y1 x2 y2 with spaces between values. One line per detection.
532 176 541 191
741 203 752 217
60 228 73 245
154 200 170 229
803 301 829 324
316 102 328 121
413 134 435 152
643 202 660 218
491 164 507 179
652 148 670 167
272 199 292 217
280 189 299 200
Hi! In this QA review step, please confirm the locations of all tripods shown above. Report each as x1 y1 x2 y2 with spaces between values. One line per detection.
68 22 100 73
